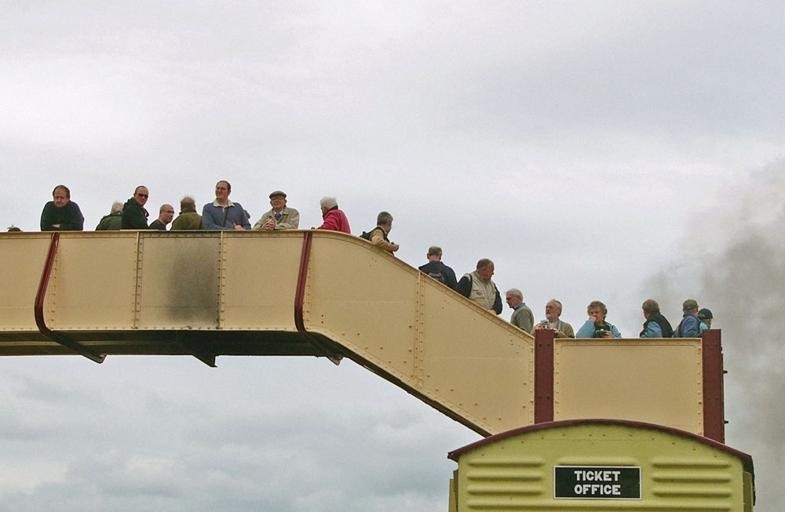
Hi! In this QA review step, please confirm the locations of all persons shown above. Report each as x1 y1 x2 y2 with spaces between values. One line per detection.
672 299 709 337
418 245 457 289
697 307 713 328
201 180 251 231
316 195 351 234
576 300 622 339
148 204 174 230
639 300 674 338
505 288 534 335
6 225 22 232
169 196 202 230
529 298 574 338
253 191 299 231
95 201 124 230
453 258 503 317
121 185 150 229
39 184 84 231
358 211 399 256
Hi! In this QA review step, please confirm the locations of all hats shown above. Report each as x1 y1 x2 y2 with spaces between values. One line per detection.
698 309 712 319
683 299 697 311
269 191 286 198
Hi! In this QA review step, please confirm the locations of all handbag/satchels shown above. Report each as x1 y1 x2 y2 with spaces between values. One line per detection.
359 227 385 240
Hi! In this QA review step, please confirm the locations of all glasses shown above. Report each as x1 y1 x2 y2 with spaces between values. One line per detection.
138 193 148 198
